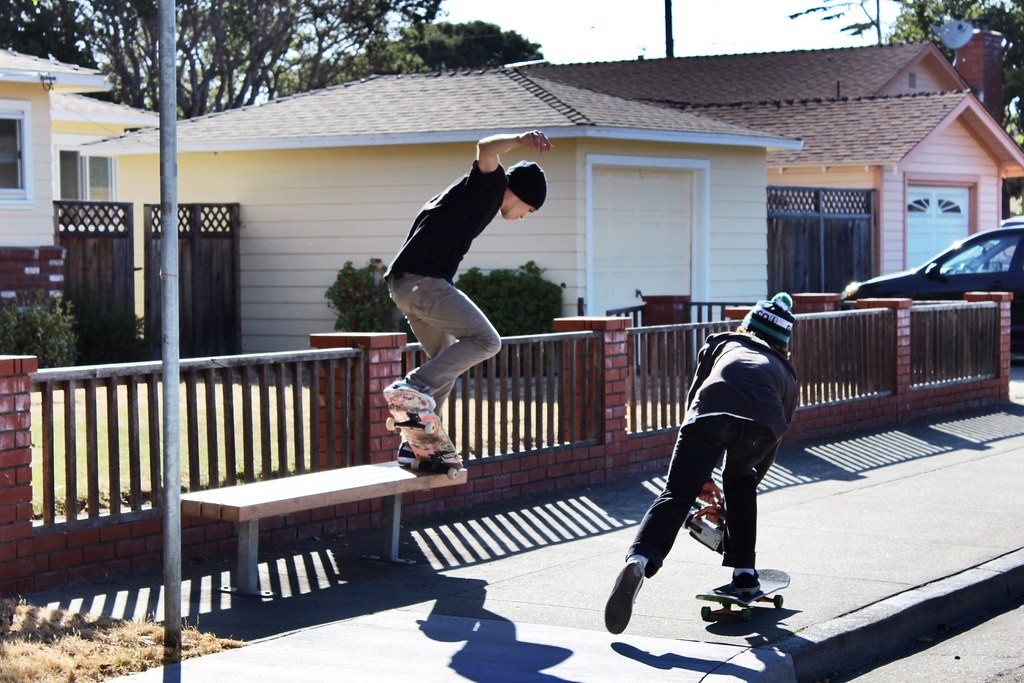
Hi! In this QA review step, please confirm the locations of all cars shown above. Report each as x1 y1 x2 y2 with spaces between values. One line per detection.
839 224 1024 348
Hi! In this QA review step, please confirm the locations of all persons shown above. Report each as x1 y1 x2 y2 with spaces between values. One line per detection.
382 130 555 468
604 290 799 634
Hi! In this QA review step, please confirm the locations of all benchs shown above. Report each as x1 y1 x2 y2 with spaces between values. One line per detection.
180 459 469 599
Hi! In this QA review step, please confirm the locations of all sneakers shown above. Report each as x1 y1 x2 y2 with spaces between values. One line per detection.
382 381 436 411
604 558 644 635
397 444 418 466
733 568 761 596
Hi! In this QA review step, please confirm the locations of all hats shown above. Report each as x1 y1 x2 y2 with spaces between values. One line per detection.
743 291 796 349
506 159 548 211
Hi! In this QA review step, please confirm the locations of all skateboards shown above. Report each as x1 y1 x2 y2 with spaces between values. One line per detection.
386 388 463 480
695 569 790 621
